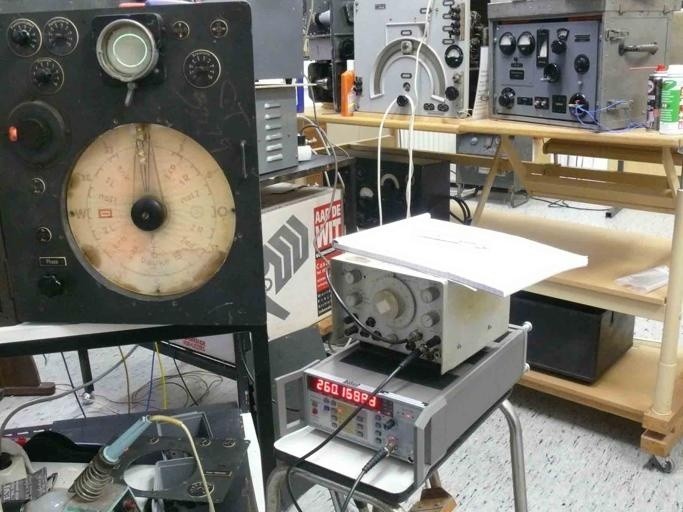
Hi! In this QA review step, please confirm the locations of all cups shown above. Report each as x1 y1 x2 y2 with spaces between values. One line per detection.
296 146 318 163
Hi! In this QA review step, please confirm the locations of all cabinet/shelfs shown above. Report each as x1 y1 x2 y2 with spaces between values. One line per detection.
298 108 681 456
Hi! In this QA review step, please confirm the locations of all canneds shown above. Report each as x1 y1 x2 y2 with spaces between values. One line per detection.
647 73 682 133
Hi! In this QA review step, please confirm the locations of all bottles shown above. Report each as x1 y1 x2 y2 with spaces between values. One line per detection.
645 63 680 134
340 59 356 117
